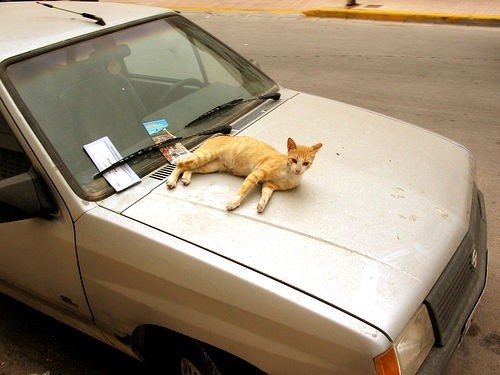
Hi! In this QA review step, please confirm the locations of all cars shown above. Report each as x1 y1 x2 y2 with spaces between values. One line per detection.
0 0 490 375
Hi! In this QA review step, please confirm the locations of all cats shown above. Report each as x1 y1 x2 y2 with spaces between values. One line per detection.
166 135 323 213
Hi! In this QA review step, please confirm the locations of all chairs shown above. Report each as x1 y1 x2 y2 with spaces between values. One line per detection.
58 72 149 157
